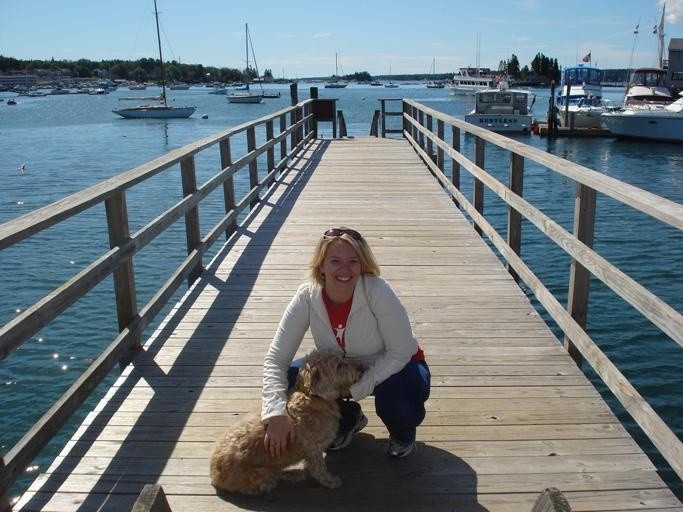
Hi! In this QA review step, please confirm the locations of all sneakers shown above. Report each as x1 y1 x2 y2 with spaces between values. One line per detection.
327 405 368 450
385 432 415 459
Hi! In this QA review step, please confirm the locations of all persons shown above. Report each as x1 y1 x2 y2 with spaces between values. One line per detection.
260 225 432 456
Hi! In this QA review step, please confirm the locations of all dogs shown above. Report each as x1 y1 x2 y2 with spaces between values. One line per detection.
209 347 365 497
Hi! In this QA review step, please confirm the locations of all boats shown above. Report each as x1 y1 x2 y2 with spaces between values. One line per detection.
425 56 445 88
0 0 280 119
370 80 383 86
551 64 621 128
465 83 536 134
450 32 512 96
601 0 682 141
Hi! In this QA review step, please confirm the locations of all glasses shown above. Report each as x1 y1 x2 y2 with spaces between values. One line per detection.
323 228 362 240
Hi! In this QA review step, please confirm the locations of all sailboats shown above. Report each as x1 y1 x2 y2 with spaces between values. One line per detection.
324 52 347 88
384 63 398 87
